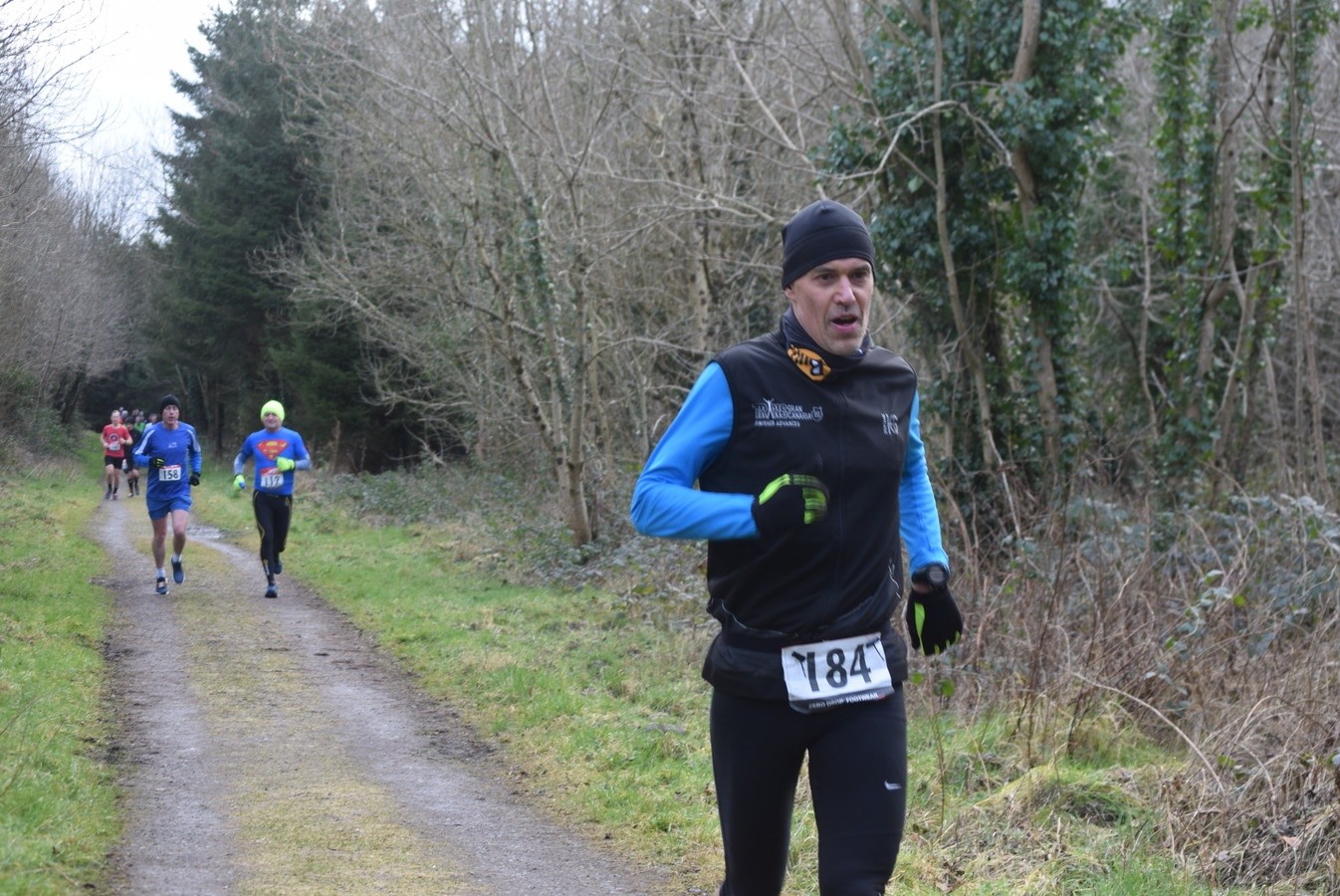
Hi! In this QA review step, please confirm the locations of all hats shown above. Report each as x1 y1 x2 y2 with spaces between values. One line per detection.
160 394 181 412
125 417 135 424
783 197 880 291
261 399 285 420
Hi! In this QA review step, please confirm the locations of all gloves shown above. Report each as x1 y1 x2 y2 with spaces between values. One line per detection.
906 586 963 658
232 475 246 490
752 472 829 533
150 457 165 467
189 473 199 486
276 457 295 472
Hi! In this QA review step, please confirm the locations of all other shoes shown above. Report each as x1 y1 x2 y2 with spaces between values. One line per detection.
129 493 133 498
113 493 117 500
135 484 140 496
106 490 111 499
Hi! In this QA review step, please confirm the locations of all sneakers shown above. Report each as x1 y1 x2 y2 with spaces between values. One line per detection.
171 554 184 582
264 584 278 598
273 556 282 574
156 576 169 595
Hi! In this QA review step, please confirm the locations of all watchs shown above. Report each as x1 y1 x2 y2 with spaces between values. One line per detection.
911 562 949 589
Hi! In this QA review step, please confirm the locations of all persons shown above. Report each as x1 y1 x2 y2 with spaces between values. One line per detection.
627 197 964 896
99 396 202 594
234 400 312 598
